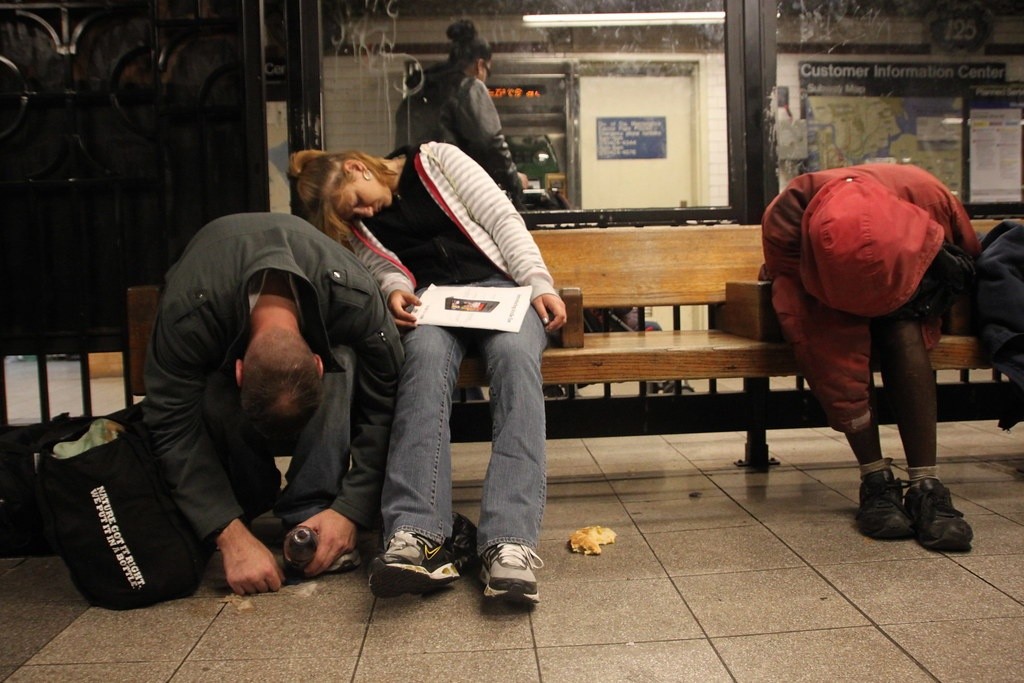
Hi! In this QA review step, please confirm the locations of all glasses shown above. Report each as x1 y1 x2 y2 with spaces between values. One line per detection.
483 63 492 79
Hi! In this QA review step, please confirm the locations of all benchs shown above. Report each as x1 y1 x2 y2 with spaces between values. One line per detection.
126 217 1024 472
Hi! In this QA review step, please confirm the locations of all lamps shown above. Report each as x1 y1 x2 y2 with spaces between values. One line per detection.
523 10 727 29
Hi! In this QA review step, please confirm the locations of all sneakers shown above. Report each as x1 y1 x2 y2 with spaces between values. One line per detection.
368 531 460 598
477 541 544 605
905 478 973 549
855 472 917 540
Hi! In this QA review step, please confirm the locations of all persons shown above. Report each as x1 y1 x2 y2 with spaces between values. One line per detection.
141 212 405 597
395 19 528 197
760 164 982 551
287 142 567 604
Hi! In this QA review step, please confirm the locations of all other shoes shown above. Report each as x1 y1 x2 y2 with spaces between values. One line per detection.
325 545 362 571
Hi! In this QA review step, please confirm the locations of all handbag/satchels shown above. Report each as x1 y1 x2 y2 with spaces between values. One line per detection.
32 400 216 610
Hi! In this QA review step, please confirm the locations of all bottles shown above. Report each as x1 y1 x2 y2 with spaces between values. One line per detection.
284 527 319 573
